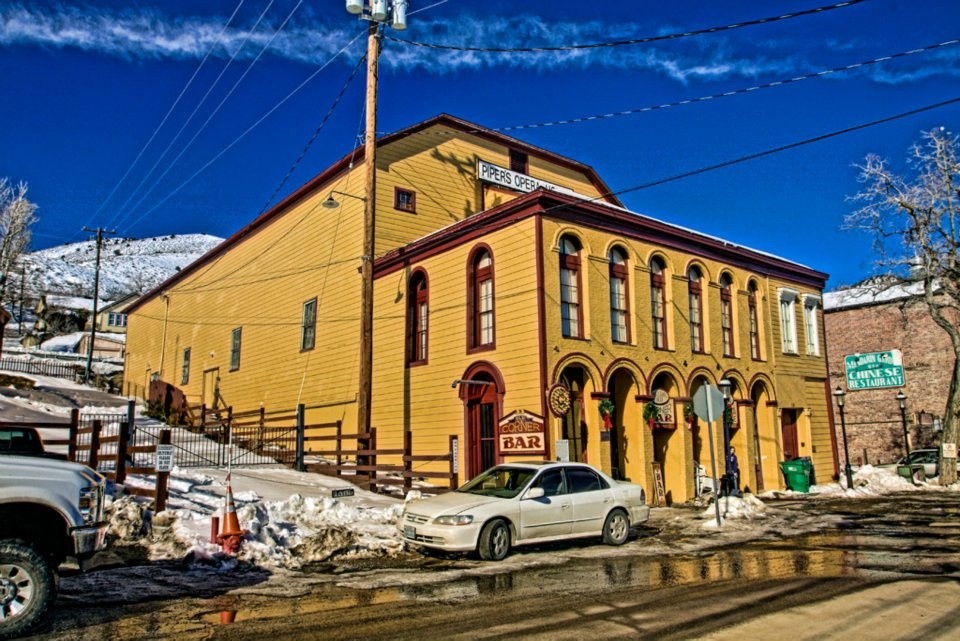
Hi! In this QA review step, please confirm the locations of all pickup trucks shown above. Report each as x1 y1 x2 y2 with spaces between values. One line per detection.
0 424 68 460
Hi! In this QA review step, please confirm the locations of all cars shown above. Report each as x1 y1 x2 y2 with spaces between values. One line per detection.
895 448 960 478
396 460 651 561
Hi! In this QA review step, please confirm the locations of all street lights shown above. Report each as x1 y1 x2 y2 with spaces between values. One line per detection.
831 385 853 488
322 191 369 473
715 377 735 492
894 390 913 482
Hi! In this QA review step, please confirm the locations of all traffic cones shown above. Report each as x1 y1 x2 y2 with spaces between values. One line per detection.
216 486 249 539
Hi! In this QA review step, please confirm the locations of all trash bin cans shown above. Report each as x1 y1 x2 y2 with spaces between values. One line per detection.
778 456 810 493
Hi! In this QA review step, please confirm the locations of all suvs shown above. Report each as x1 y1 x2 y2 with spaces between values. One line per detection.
0 460 107 639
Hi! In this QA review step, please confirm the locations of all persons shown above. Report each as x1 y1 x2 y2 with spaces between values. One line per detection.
729 447 739 491
